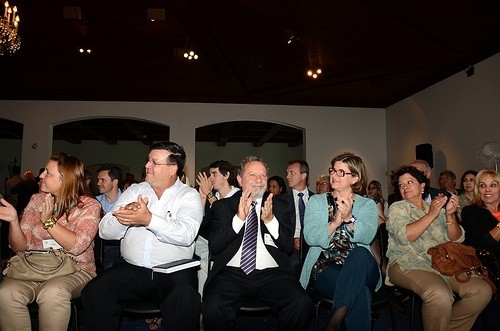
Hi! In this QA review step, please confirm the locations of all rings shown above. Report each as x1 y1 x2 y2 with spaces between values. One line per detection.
437 201 439 203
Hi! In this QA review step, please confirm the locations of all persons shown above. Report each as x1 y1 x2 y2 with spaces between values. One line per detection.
315 176 334 194
201 153 311 330
92 166 123 217
438 170 462 197
196 159 242 215
455 169 479 220
299 152 379 331
368 180 389 217
0 152 102 331
80 141 201 331
361 190 386 258
412 160 459 221
384 166 493 330
272 157 317 268
461 168 500 290
267 175 288 198
0 155 39 222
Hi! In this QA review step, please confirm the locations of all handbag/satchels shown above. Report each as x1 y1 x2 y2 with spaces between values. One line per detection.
3 247 80 281
427 241 497 293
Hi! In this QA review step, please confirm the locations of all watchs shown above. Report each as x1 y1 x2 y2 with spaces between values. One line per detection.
343 215 355 224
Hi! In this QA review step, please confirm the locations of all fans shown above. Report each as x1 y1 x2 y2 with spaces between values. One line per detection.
477 142 500 174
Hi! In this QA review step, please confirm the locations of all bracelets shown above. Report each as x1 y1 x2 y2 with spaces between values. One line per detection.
497 224 500 229
42 217 56 230
446 214 456 224
206 192 214 199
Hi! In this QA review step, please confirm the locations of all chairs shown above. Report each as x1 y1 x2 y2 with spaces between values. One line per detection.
300 220 393 331
93 215 196 331
380 224 464 331
204 240 306 330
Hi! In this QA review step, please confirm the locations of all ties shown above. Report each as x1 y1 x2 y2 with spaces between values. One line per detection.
240 201 258 275
298 193 305 238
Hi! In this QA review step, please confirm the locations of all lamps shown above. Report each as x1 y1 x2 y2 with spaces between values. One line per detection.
73 47 96 57
278 29 295 46
183 35 201 65
303 47 327 80
0 0 22 60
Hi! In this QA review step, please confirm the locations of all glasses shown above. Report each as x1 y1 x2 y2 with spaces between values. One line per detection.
145 157 175 167
329 168 353 177
